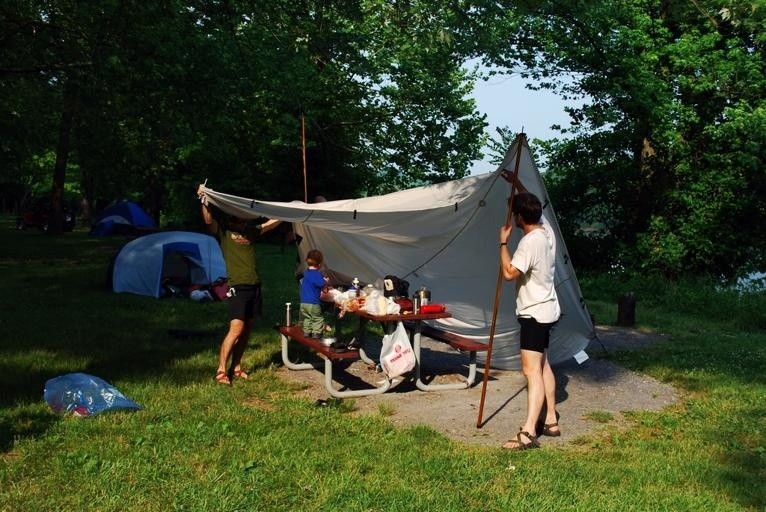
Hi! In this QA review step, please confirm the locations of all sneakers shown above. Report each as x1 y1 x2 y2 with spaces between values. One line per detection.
303 331 323 339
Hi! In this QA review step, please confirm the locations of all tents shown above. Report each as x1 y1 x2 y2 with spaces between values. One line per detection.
104 230 227 299
87 202 157 241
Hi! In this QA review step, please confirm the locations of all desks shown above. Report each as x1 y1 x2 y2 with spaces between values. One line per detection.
330 289 452 398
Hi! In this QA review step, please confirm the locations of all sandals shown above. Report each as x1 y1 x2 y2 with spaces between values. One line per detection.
536 422 560 437
501 425 540 450
229 367 249 378
214 370 231 386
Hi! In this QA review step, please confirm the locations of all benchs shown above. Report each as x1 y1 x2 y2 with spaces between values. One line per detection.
270 319 392 400
394 320 490 393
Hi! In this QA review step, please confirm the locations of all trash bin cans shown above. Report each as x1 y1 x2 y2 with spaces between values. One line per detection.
617 296 636 326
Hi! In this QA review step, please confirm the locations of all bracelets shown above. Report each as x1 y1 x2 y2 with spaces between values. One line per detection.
203 203 208 208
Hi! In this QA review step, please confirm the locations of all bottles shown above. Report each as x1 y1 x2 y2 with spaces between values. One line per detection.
366 283 374 295
350 277 360 297
412 286 431 316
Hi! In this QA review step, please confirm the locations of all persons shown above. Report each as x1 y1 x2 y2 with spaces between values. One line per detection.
300 250 329 339
295 196 328 280
80 194 92 227
499 168 562 452
196 184 282 387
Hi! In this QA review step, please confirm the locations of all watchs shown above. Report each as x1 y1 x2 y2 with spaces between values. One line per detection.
500 243 507 248
